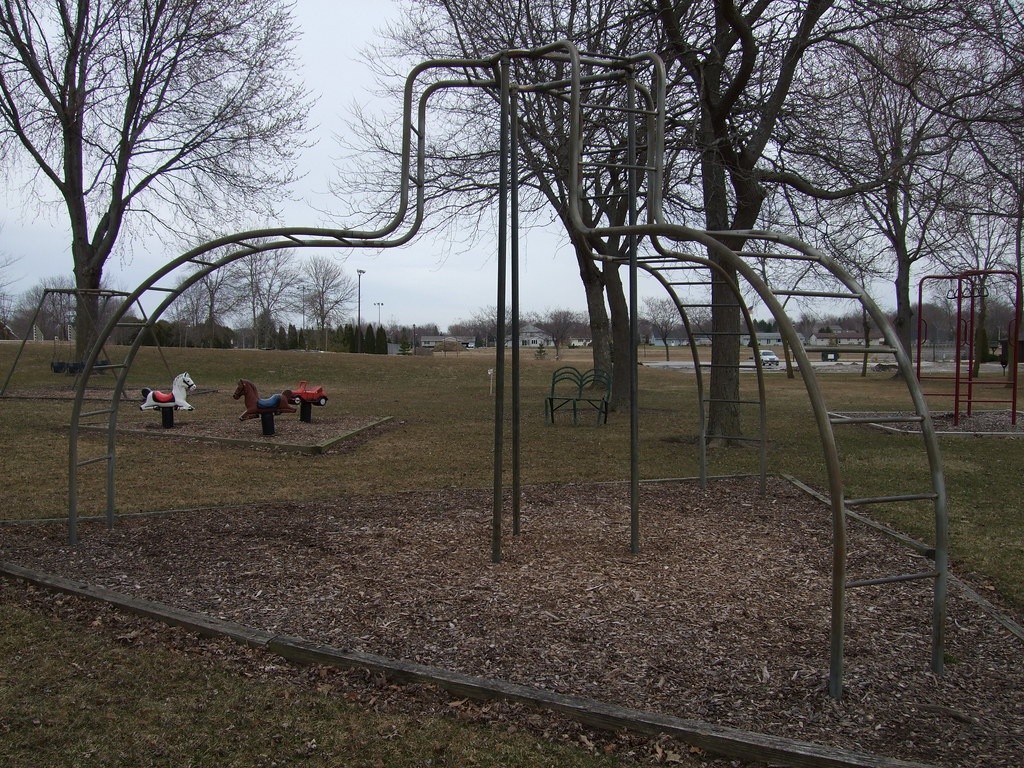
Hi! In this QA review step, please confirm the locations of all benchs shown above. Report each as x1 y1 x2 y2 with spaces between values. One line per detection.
545 366 613 425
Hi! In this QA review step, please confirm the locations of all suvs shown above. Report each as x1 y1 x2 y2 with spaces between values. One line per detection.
287 381 328 406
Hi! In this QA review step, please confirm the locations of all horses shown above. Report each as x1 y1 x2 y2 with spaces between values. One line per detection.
232 378 297 421
140 371 197 411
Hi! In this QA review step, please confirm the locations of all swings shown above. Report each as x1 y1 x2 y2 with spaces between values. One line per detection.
50 292 109 376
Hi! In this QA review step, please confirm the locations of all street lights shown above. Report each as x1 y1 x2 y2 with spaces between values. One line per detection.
296 285 312 333
356 269 366 354
413 324 417 355
373 302 384 327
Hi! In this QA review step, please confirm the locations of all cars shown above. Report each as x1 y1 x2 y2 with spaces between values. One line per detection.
760 350 781 367
